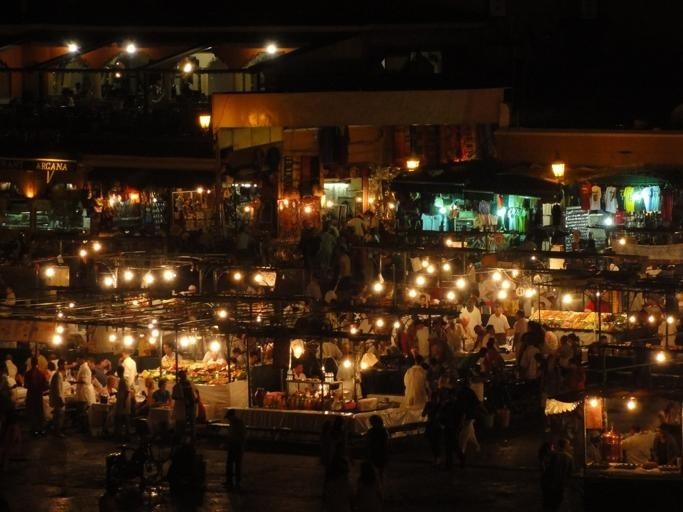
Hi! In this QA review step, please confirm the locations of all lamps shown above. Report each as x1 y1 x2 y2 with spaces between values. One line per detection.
550 157 567 181
195 105 211 133
406 147 421 173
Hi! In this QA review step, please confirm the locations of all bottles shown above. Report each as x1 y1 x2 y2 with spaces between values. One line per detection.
254 387 325 409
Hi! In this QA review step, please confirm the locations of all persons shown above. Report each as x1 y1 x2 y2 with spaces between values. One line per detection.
1 182 682 512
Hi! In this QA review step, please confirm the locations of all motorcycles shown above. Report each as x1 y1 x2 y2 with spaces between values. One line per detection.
101 439 149 496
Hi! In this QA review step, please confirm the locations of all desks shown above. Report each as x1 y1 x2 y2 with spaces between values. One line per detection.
1 356 249 436
219 393 428 494
